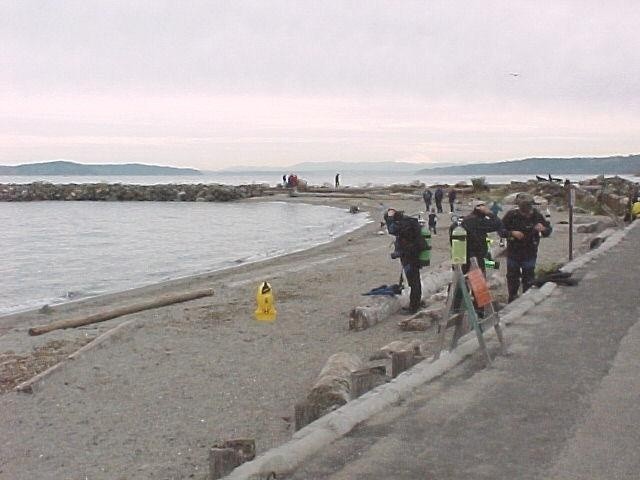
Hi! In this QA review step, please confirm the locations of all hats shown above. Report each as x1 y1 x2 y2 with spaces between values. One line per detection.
473 200 485 212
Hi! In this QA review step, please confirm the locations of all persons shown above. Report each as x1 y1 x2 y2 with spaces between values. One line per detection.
422 187 433 211
283 174 297 188
450 192 552 318
445 189 456 212
428 208 437 234
335 173 339 187
383 208 430 314
435 187 443 213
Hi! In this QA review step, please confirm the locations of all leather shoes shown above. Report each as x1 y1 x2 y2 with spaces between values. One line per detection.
400 307 417 315
